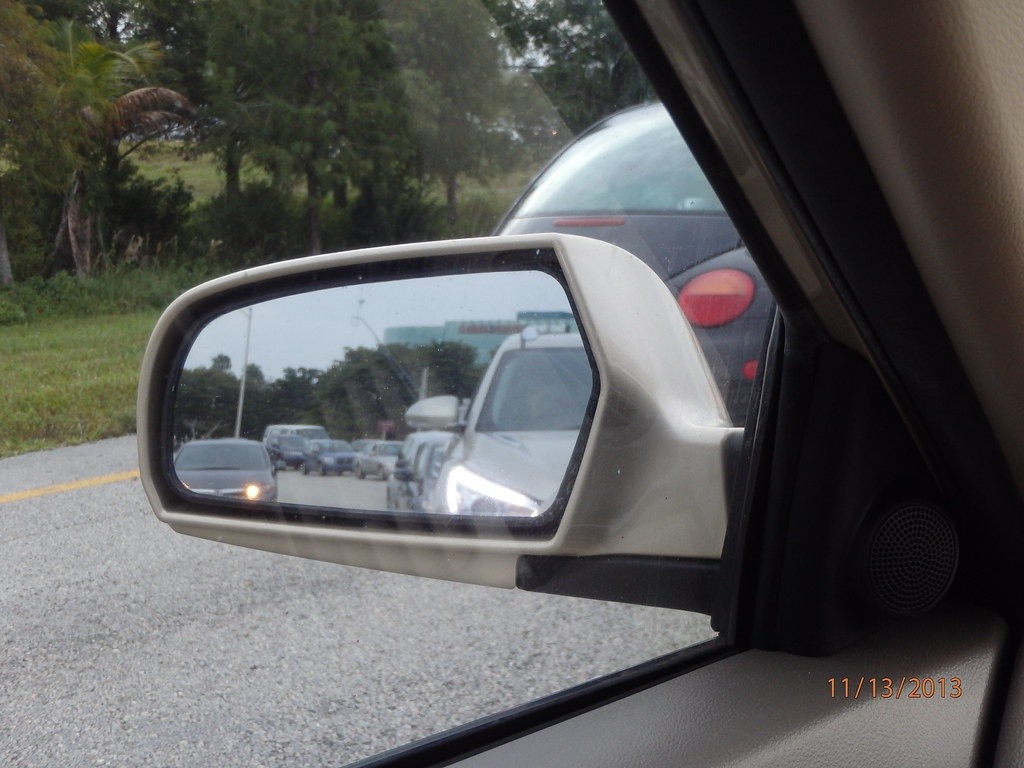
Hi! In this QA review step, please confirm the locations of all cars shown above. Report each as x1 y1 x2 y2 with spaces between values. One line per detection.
172 437 288 502
302 437 354 472
358 430 460 513
263 434 306 471
490 95 774 426
390 322 594 517
353 440 404 482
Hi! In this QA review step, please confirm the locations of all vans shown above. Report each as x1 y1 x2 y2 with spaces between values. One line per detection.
263 424 331 440
